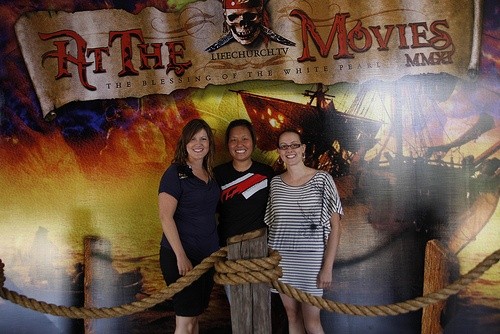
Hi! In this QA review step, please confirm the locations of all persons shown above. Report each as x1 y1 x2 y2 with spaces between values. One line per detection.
263 128 345 334
159 119 221 333
211 118 289 334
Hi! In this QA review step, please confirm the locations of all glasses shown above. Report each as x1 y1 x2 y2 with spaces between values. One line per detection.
280 143 303 150
